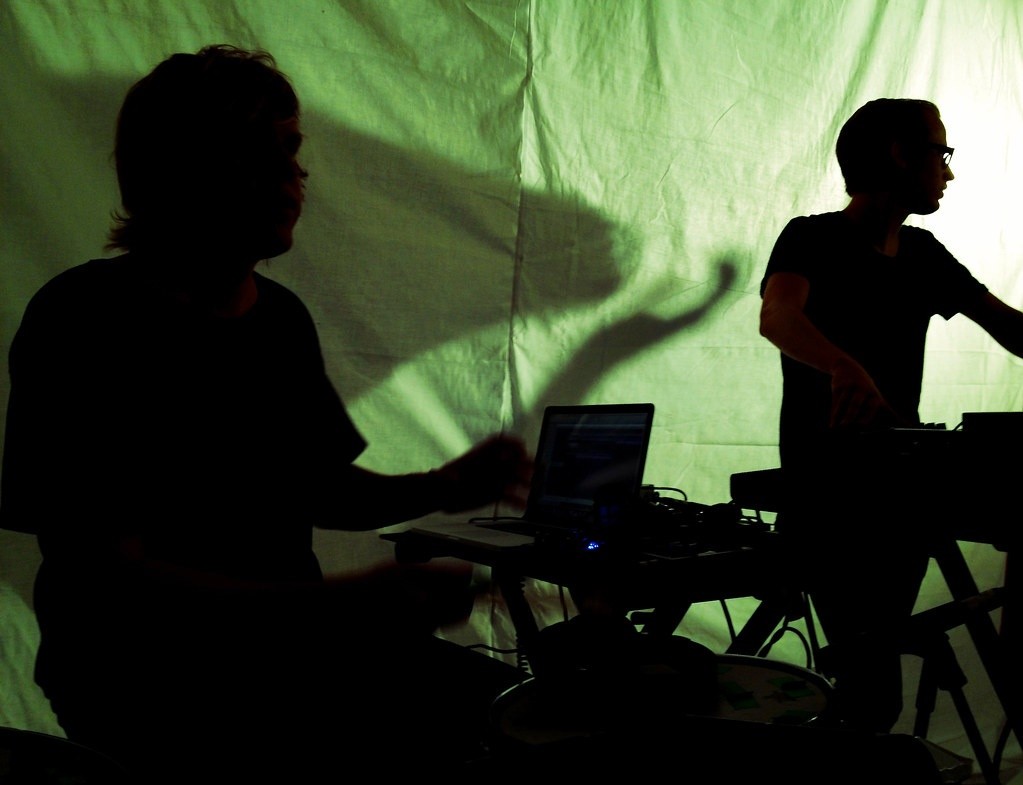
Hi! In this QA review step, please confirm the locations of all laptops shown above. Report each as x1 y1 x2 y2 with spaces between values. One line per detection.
411 404 654 551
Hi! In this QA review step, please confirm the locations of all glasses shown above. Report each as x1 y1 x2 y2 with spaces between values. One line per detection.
929 146 956 168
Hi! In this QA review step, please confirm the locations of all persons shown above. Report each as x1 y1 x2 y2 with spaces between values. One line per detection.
3 43 534 754
759 98 1021 732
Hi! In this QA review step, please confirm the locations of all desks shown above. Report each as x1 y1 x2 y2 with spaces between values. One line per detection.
377 522 545 677
